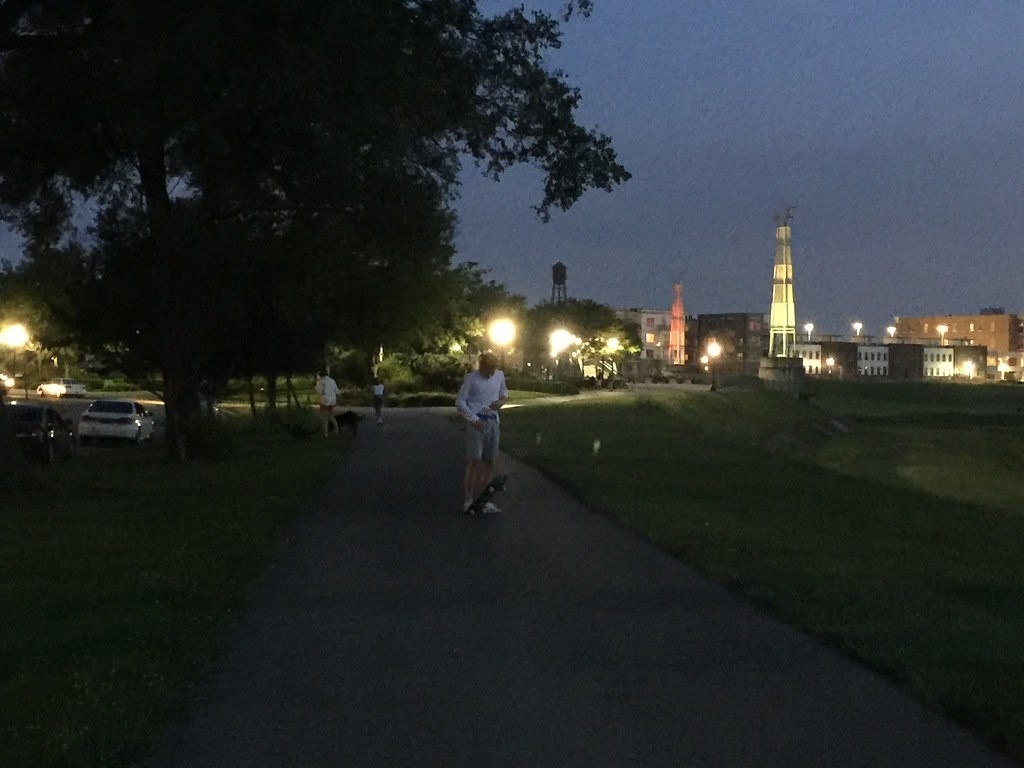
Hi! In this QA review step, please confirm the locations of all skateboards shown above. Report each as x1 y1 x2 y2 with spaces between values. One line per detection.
465 473 509 516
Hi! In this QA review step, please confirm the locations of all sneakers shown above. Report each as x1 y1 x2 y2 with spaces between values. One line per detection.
463 500 475 514
481 501 501 514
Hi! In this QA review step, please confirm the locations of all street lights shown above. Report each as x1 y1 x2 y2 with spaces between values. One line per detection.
606 337 618 390
937 325 948 345
826 357 834 382
489 318 517 378
550 329 573 382
701 343 722 391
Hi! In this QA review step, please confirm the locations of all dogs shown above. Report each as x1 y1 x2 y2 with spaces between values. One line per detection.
318 410 366 436
279 423 318 439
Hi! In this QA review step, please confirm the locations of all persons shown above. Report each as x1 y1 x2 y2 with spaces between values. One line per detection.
313 369 341 439
455 353 508 515
371 377 384 424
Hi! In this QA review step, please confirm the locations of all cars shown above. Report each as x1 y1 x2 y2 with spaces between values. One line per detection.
36 378 87 399
78 399 154 446
6 405 77 463
